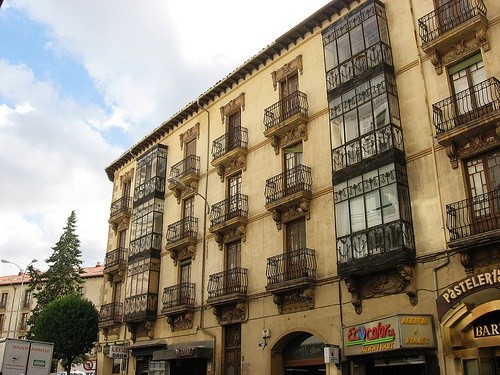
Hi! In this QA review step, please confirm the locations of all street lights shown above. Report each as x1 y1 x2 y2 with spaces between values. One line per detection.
0 257 38 340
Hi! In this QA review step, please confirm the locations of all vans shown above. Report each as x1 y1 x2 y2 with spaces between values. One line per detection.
59 371 89 375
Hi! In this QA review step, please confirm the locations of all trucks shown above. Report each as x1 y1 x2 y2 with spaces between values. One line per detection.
0 336 54 375
